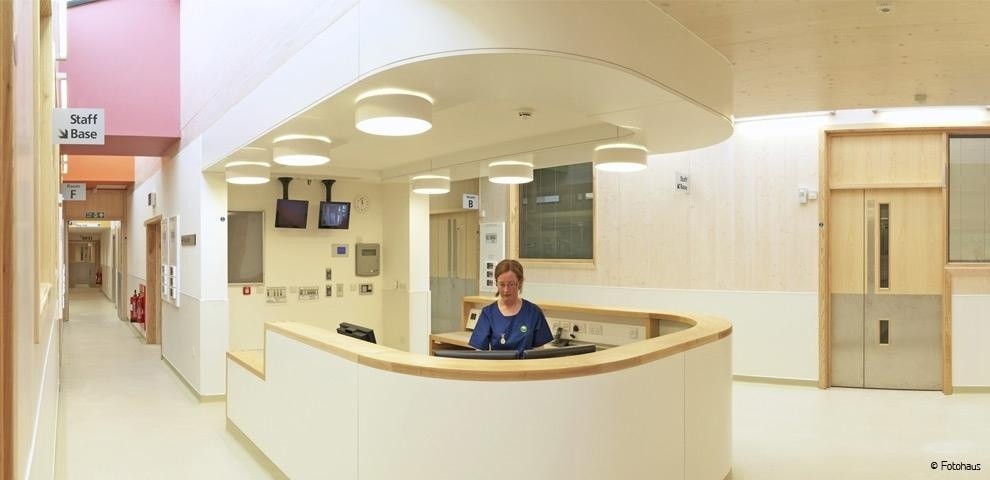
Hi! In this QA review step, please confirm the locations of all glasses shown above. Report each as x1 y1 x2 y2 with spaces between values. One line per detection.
498 280 520 288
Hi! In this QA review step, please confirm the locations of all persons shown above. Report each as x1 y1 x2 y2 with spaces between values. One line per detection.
469 258 553 360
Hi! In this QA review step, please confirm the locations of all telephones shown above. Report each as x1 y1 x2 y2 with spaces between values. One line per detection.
552 328 569 347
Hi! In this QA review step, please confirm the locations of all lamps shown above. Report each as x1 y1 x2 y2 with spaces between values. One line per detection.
353 92 435 136
272 139 330 167
410 159 451 195
590 127 649 175
224 161 270 185
488 161 534 185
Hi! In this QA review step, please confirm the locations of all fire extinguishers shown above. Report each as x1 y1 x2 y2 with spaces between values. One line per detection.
130 290 144 323
95 270 102 284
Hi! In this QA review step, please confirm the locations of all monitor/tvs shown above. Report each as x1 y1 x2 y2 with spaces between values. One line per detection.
318 200 350 230
523 344 597 359
275 199 308 229
435 350 520 359
337 322 377 344
